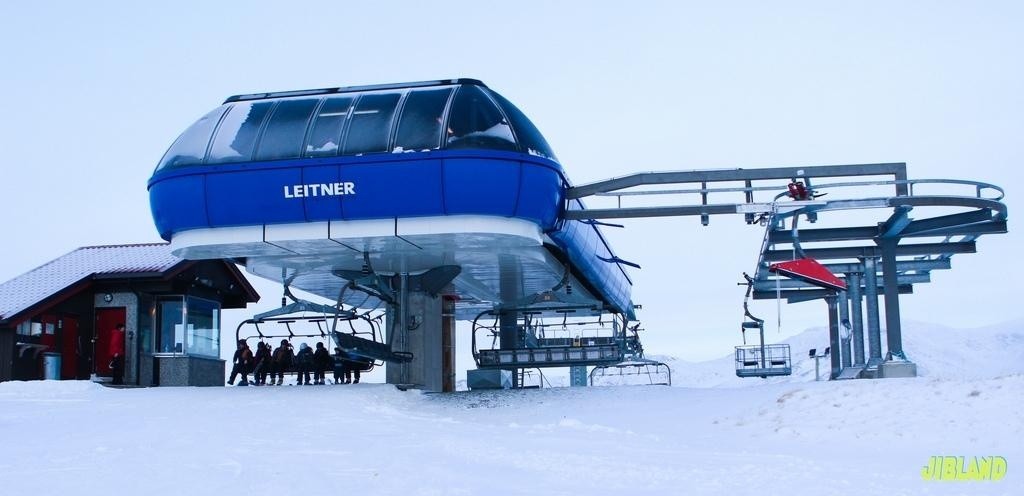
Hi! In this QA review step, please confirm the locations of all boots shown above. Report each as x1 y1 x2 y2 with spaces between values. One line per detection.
335 378 359 384
255 378 283 385
297 378 325 385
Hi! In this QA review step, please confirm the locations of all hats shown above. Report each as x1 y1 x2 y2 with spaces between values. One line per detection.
317 342 323 348
239 339 246 345
258 342 264 348
282 340 288 345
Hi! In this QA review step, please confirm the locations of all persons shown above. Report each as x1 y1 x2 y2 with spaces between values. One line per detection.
269 339 289 385
346 359 361 384
253 342 271 385
109 323 126 383
227 339 253 386
295 342 313 385
330 348 346 384
313 342 329 385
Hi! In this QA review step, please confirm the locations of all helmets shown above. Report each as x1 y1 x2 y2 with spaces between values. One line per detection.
300 343 307 349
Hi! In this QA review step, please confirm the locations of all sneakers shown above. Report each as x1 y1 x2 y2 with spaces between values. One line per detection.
227 380 233 385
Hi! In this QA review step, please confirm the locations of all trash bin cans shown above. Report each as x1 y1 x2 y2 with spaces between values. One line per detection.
38 351 62 380
